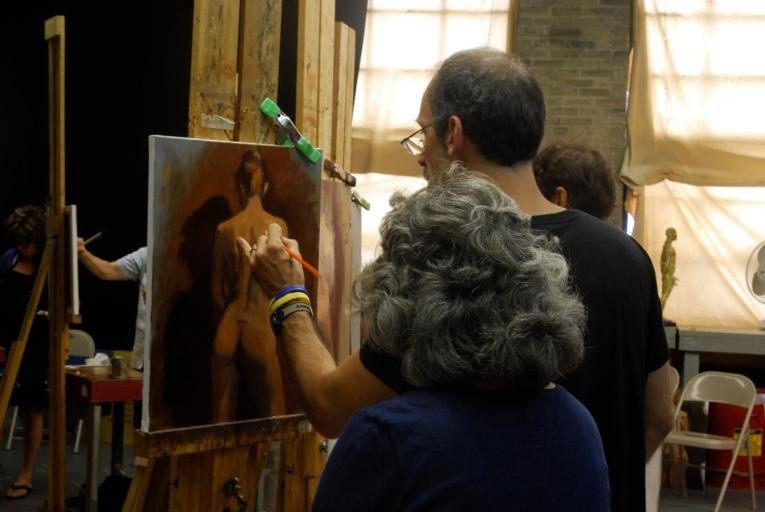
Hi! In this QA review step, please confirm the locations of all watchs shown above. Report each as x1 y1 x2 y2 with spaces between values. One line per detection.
271 303 314 336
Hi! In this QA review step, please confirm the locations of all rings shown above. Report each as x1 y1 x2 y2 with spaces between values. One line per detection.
250 247 257 253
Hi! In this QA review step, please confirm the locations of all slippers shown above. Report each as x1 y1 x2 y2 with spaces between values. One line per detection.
4 484 32 499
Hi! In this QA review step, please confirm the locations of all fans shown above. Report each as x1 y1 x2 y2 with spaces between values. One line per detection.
745 239 765 331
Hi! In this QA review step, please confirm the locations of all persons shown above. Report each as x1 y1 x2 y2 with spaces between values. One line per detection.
75 233 148 362
1 206 49 499
313 162 613 512
237 49 675 512
211 149 288 428
532 143 616 221
661 227 679 314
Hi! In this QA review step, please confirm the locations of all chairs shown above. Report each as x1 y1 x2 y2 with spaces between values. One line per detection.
662 371 757 512
5 330 95 454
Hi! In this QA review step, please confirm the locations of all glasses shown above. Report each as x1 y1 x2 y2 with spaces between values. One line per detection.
401 120 437 156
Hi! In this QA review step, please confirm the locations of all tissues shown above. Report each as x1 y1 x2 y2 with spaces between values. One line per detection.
78 353 114 378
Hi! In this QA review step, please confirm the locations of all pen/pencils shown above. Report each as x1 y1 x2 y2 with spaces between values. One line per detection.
82 232 102 245
262 228 322 279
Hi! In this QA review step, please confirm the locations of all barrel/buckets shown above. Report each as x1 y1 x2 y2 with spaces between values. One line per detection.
706 386 765 489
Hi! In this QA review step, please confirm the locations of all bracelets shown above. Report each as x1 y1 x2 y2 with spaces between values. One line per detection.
267 285 310 321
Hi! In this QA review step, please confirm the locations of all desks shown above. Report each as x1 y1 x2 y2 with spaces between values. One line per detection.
65 364 142 511
